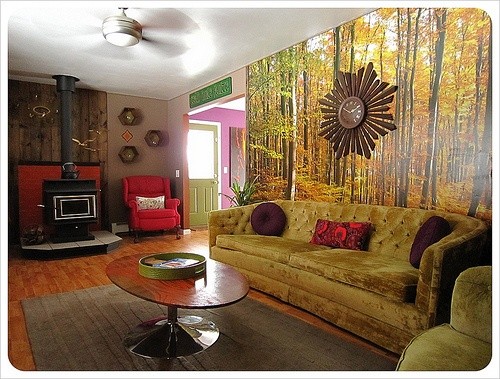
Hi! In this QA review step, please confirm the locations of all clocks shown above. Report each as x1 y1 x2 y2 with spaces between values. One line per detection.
318 62 397 160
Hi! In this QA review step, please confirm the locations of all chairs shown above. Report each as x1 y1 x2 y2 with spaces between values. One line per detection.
123 175 182 243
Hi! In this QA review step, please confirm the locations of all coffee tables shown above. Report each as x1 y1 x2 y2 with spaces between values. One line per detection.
106 253 249 359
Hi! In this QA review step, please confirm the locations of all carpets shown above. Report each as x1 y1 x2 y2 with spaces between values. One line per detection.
19 286 398 371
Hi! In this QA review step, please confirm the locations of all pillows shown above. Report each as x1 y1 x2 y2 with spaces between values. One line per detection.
310 219 372 251
136 196 165 210
409 216 450 269
251 203 286 236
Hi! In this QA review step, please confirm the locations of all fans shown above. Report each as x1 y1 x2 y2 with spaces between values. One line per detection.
42 8 202 63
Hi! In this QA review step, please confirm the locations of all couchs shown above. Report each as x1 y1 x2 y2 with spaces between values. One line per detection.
207 200 488 355
395 266 492 371
